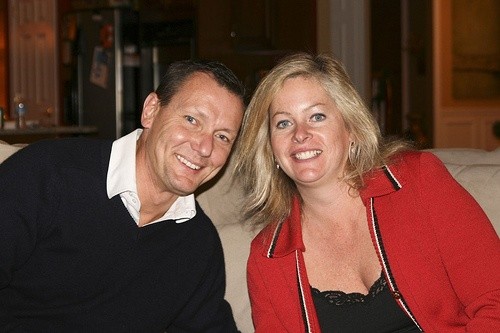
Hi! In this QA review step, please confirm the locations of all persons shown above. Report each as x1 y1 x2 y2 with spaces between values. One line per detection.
0 59 246 333
217 51 500 333
